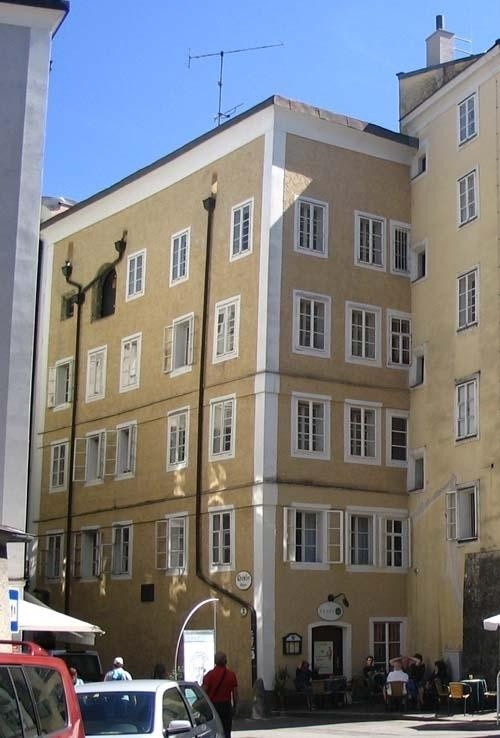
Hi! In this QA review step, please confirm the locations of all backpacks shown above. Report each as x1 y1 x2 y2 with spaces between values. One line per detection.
112 669 126 680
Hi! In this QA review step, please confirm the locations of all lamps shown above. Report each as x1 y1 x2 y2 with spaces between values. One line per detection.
328 593 349 608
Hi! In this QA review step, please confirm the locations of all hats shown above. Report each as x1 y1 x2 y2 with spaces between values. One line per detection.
114 657 123 664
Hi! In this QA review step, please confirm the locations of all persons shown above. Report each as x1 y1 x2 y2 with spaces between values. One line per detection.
153 664 165 678
103 657 133 681
294 654 448 710
69 667 84 685
200 652 239 738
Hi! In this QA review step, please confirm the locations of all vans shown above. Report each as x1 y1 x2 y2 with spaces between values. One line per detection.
0 641 105 738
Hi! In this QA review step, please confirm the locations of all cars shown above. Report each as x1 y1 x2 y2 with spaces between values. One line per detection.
76 678 227 737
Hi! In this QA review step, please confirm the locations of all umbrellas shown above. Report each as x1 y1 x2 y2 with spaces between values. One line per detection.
483 614 500 631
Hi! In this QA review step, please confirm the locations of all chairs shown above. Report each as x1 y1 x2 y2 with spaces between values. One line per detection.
296 671 497 716
